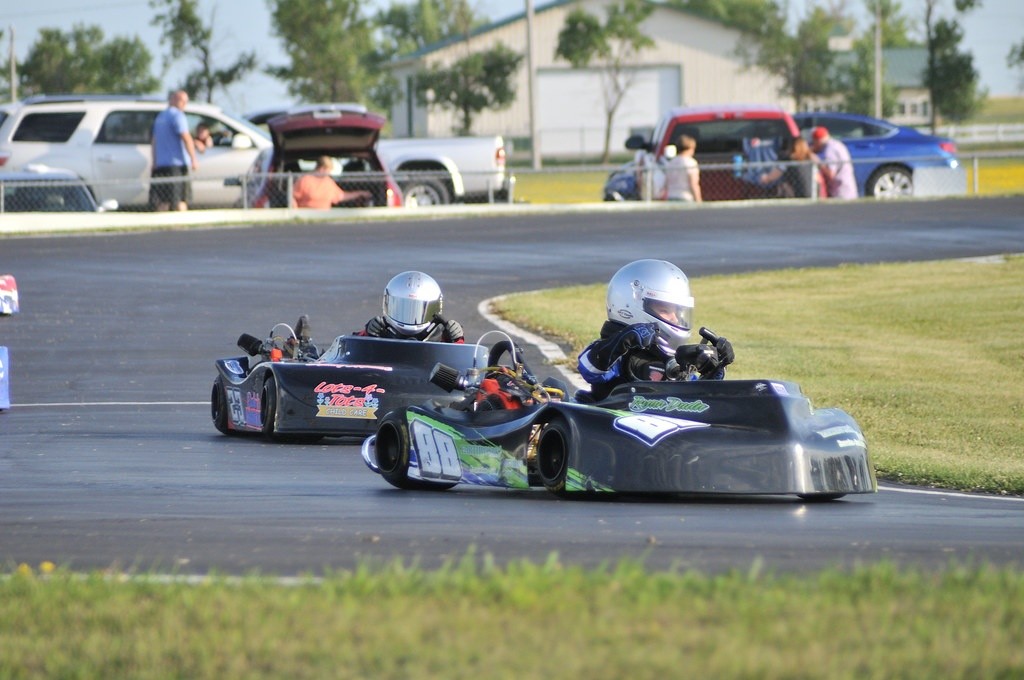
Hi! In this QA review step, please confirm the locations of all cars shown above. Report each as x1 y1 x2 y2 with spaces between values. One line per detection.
624 102 828 202
0 94 273 212
601 111 966 204
223 105 404 209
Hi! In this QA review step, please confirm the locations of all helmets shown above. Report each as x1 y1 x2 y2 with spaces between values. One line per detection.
605 258 694 356
382 271 443 337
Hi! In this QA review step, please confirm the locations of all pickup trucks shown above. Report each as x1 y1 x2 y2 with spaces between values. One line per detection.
205 103 507 206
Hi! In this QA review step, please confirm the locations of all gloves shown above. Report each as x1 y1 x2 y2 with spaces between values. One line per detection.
366 316 386 337
602 320 658 363
713 337 734 366
446 319 463 341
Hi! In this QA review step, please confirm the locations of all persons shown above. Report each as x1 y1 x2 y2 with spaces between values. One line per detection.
578 258 736 403
193 123 213 151
660 134 701 201
358 271 465 344
758 136 827 198
149 90 198 210
292 155 372 208
807 126 859 200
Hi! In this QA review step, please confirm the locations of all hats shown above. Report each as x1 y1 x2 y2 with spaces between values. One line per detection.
810 125 829 142
